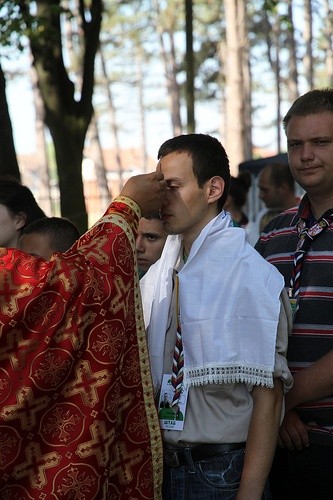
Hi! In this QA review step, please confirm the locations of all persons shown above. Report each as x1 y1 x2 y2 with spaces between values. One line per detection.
222 173 259 248
20 217 80 261
0 182 48 248
138 133 293 500
0 171 169 500
254 88 333 500
258 160 302 232
134 212 168 273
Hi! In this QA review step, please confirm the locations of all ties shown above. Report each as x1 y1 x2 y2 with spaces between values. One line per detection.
166 273 184 409
285 216 332 319
